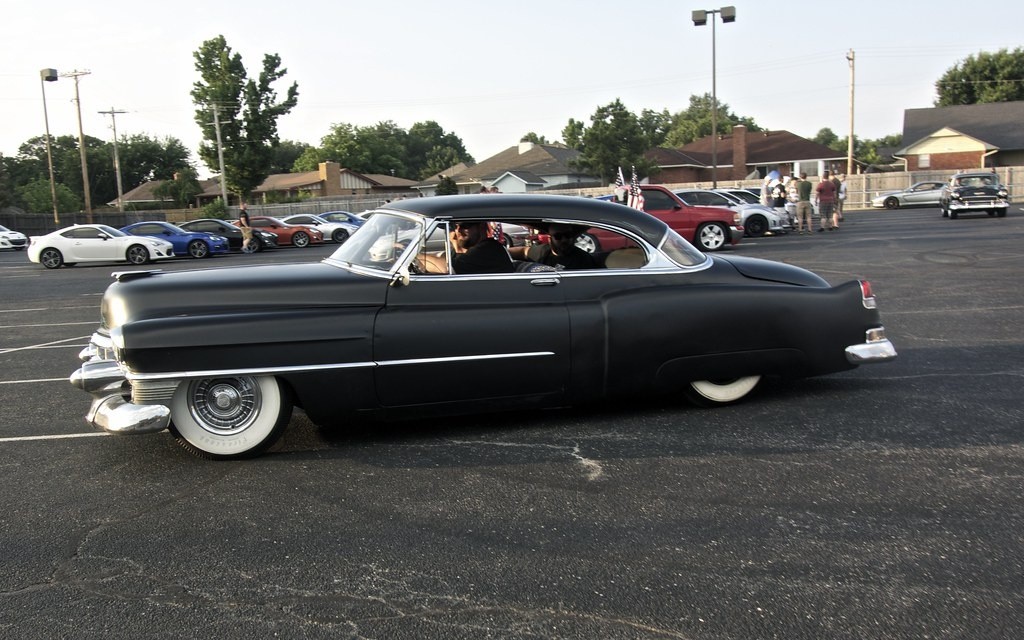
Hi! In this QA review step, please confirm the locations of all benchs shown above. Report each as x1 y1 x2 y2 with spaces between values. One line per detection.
603 248 645 270
510 259 558 272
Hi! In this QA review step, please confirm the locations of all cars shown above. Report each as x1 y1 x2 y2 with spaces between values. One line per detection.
178 219 279 253
119 221 231 259
28 223 175 269
281 213 359 243
939 172 1010 220
673 187 814 238
0 225 28 251
319 210 377 227
871 180 948 209
69 192 897 462
231 216 324 248
362 218 529 263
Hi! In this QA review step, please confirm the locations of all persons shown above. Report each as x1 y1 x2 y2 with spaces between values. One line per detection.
759 170 847 236
991 165 996 174
537 221 608 271
931 186 936 189
393 221 514 274
956 179 966 186
964 180 970 185
239 202 253 253
979 178 990 185
477 186 500 193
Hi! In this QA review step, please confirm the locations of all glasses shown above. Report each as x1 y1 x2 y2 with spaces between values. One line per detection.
449 223 474 231
550 232 574 240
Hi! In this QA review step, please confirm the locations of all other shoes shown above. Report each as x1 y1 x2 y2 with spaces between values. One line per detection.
829 227 833 232
818 228 826 232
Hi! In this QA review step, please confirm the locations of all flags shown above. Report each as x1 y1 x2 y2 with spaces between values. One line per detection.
614 168 626 202
492 221 504 244
627 166 645 212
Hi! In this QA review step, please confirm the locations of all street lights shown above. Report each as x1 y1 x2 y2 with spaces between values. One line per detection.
691 6 737 189
40 69 60 231
845 49 856 202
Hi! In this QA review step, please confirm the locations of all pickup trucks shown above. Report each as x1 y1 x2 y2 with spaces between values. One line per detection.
539 185 746 258
